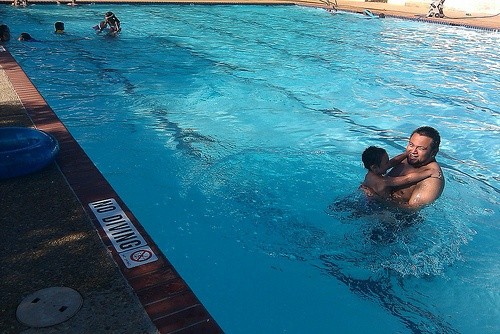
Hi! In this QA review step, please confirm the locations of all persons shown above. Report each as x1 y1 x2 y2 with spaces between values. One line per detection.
0 24 32 42
14 0 29 7
360 126 446 202
361 145 442 198
55 21 65 33
98 12 121 38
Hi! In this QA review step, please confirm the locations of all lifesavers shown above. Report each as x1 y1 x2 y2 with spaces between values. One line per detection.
0 126 59 178
